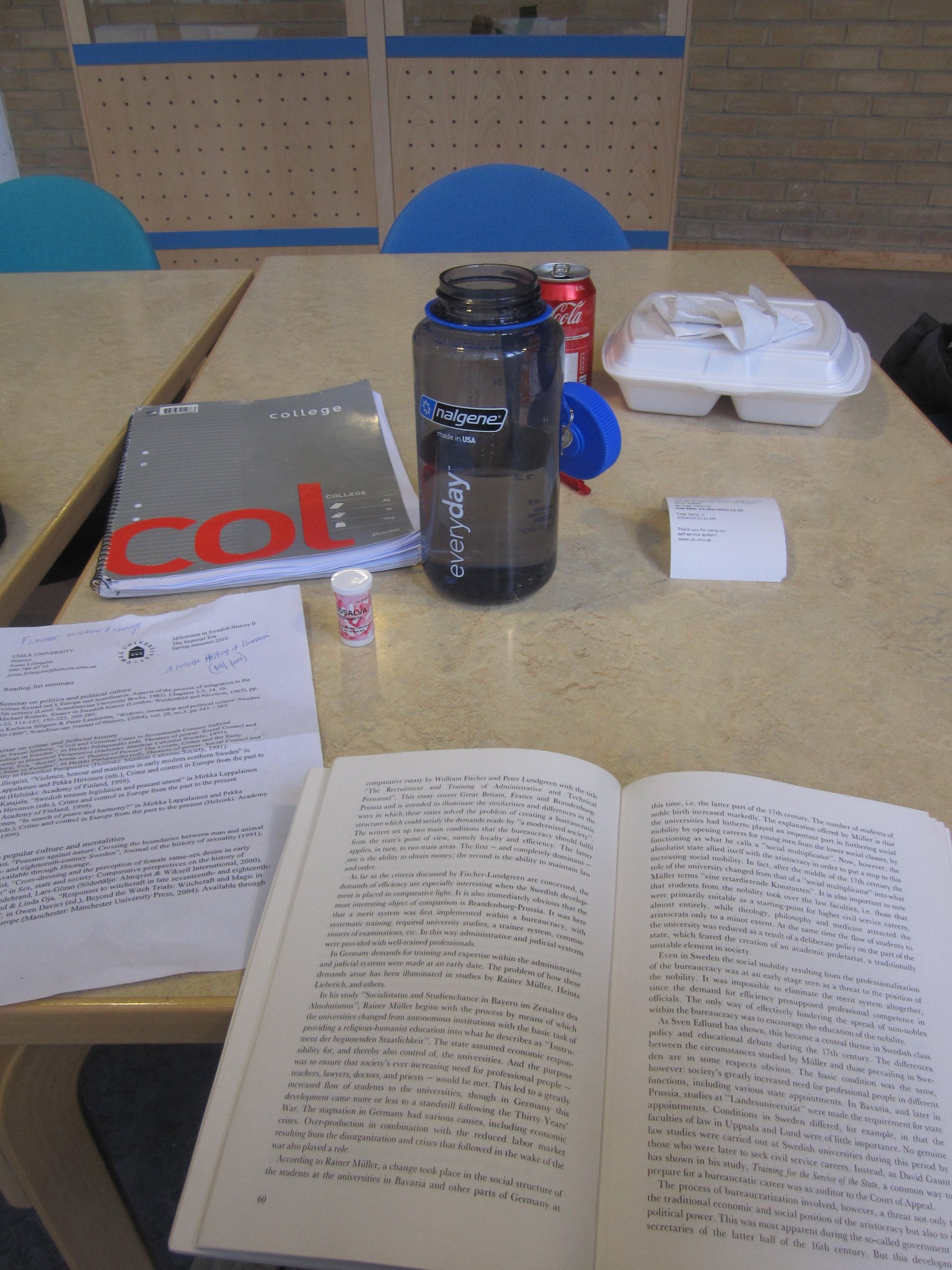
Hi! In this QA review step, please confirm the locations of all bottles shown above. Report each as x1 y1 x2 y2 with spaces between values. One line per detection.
331 567 374 647
412 263 565 607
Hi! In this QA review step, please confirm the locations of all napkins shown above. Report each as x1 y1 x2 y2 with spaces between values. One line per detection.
651 285 815 351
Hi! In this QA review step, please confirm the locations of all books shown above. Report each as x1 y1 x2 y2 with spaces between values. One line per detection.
166 745 952 1270
89 378 421 600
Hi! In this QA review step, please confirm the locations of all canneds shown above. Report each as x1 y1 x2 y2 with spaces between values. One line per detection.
530 261 596 389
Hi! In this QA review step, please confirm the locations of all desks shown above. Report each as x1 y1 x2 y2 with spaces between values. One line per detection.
1 251 952 1270
1 267 256 630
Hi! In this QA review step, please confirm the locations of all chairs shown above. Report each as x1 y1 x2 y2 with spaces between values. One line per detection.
0 176 161 273
379 161 630 254
